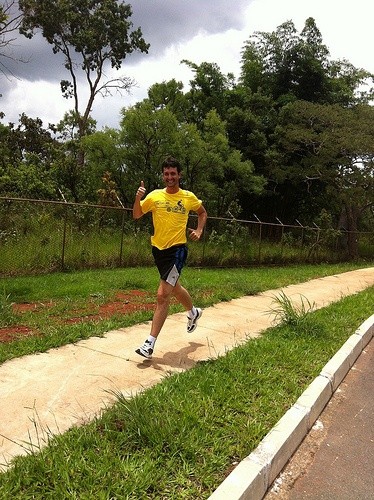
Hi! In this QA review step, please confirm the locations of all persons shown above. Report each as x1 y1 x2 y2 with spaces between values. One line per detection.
127 161 208 361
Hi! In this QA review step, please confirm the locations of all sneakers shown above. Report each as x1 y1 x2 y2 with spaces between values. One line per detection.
136 339 155 359
187 306 201 333
